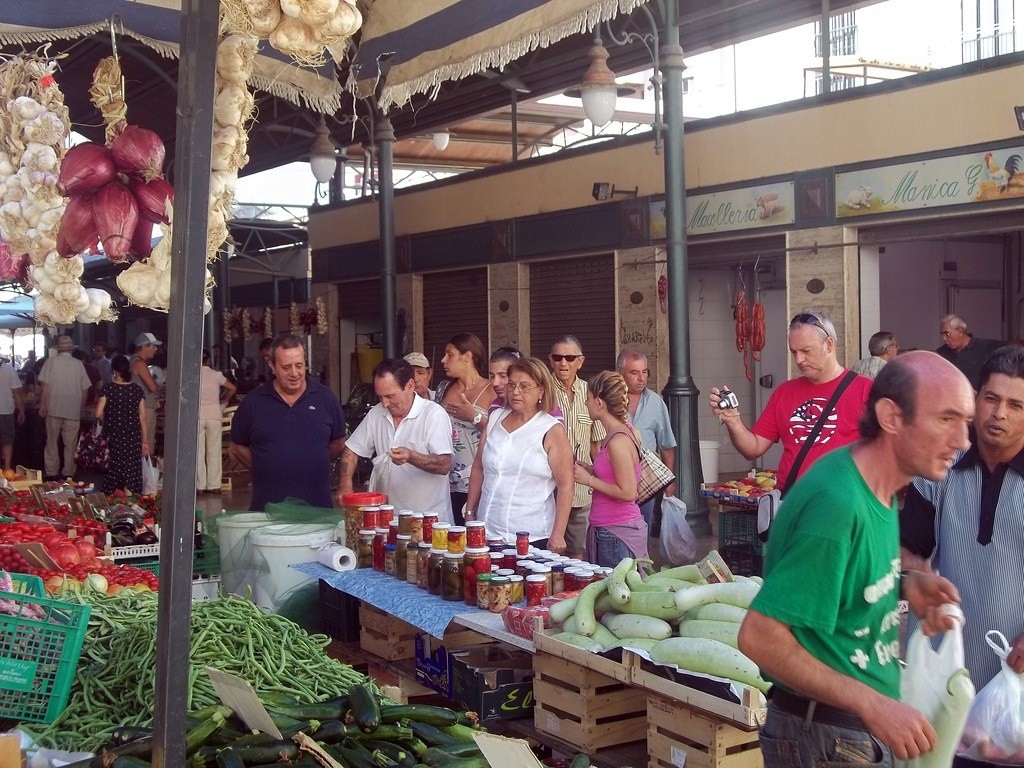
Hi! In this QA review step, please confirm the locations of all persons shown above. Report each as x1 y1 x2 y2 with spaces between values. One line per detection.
617 351 677 554
899 343 1024 768
197 344 238 494
228 333 346 513
573 370 652 580
402 332 564 527
738 351 976 768
334 357 456 526
935 315 1009 395
549 334 608 561
709 311 876 505
465 357 573 555
851 331 898 379
0 333 167 497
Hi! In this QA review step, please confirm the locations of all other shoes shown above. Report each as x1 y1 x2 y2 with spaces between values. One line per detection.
198 489 220 497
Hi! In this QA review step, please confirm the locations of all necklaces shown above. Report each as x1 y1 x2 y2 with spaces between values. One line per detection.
458 377 480 400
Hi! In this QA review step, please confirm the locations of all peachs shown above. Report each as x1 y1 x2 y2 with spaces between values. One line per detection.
0 467 28 482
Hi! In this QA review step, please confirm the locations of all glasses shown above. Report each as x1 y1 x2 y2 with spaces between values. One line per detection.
92 349 103 352
790 314 830 337
503 383 541 393
497 347 522 359
550 353 583 362
941 330 956 337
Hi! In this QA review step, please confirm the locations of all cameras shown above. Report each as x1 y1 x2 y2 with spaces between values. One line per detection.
718 390 740 410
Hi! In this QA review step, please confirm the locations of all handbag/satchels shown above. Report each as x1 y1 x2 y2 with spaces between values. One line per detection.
24 392 34 400
141 455 159 495
368 452 392 495
627 425 676 508
74 419 109 470
955 631 1024 767
658 493 699 568
892 604 976 768
756 489 783 543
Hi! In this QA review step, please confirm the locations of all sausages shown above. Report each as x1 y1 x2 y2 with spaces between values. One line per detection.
734 288 766 383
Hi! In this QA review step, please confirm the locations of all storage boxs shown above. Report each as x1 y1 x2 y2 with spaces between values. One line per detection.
0 465 221 724
318 576 768 768
719 511 767 580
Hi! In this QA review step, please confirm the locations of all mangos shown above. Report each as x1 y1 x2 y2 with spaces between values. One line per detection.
708 470 775 498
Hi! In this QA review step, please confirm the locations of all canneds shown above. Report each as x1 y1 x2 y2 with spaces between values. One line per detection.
357 503 612 612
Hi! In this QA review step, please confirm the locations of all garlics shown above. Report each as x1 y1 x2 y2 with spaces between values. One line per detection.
209 0 363 261
0 95 116 324
222 298 327 342
114 229 172 312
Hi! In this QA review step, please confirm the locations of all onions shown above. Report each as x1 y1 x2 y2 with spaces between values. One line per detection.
55 126 174 262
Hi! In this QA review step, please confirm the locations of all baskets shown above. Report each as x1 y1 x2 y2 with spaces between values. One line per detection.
717 511 763 556
109 533 222 578
0 570 92 725
718 548 763 577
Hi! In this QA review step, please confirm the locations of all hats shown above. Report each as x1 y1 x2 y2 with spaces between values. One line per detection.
53 336 80 350
402 352 430 367
136 332 162 347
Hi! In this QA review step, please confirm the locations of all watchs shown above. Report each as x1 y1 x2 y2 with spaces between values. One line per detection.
472 413 482 425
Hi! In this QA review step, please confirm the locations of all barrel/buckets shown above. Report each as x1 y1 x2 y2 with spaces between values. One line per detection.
215 510 336 617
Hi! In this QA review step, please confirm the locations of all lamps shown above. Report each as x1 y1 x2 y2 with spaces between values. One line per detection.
432 126 451 151
592 183 638 201
579 25 619 130
309 113 337 184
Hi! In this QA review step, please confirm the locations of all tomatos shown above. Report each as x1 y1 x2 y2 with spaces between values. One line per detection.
0 486 108 597
101 562 159 596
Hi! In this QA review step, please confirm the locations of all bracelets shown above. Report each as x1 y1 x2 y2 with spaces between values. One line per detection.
465 511 475 516
588 476 594 495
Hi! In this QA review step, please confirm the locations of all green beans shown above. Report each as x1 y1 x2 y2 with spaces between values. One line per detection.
0 568 409 760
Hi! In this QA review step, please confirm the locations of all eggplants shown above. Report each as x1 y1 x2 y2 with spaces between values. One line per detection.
103 521 158 546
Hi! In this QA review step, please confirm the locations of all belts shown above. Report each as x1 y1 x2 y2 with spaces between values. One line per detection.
768 687 871 732
